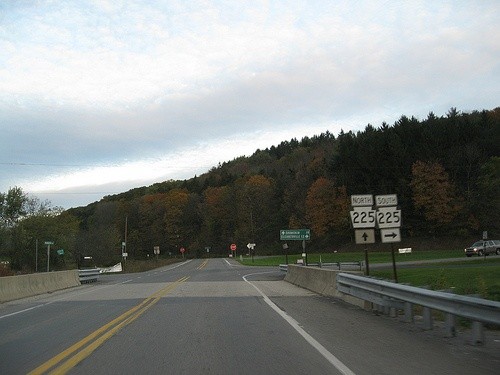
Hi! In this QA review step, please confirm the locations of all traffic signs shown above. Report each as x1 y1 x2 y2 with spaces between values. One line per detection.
279 229 311 240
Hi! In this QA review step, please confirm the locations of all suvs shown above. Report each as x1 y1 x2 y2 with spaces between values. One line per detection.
464 239 500 257
